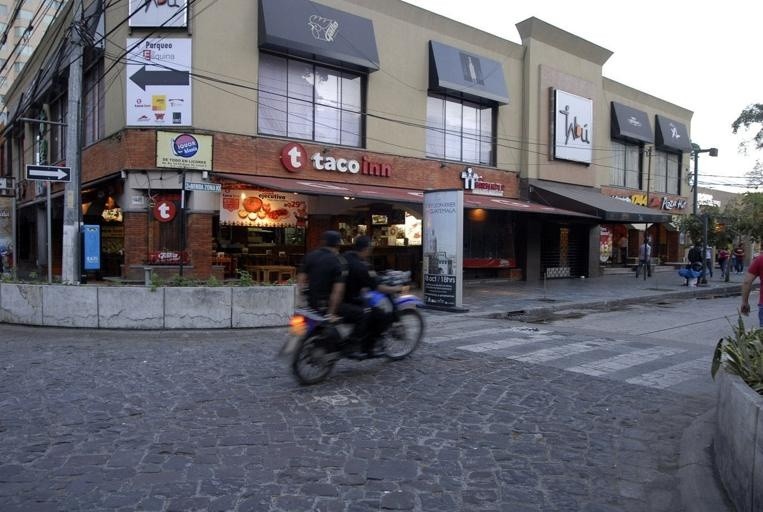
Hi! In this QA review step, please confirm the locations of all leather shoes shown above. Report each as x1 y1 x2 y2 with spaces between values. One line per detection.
346 350 387 358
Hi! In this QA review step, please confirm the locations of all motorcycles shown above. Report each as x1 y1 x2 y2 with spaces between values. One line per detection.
279 271 426 390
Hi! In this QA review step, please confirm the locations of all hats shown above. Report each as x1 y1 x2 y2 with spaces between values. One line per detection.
356 235 370 248
321 230 344 247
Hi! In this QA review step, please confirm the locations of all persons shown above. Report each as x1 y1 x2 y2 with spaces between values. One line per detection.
705 245 713 278
635 238 651 278
718 242 745 279
680 241 701 286
615 233 629 267
295 230 378 357
340 234 405 354
739 252 763 330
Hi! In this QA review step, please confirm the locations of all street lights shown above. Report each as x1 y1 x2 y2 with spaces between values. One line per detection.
689 141 719 244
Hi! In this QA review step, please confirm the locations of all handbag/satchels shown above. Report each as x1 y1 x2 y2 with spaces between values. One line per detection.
686 264 691 269
